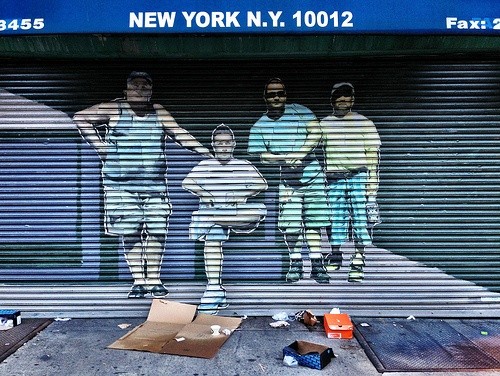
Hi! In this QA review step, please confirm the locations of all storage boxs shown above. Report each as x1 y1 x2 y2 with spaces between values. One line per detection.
1 309 22 328
323 313 354 338
283 340 338 370
